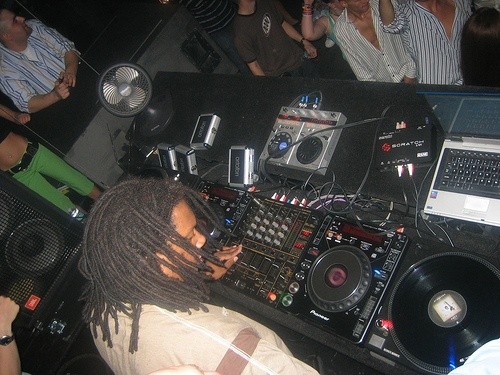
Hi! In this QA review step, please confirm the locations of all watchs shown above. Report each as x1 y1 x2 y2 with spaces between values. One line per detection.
0 333 17 347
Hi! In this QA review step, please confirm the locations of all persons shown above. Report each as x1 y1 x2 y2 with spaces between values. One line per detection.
0 104 104 225
231 0 318 76
300 0 349 70
0 294 24 375
459 6 500 86
179 0 247 76
78 176 320 375
0 8 82 112
332 0 418 82
377 0 472 85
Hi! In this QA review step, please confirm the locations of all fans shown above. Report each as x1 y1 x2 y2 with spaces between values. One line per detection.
94 61 176 137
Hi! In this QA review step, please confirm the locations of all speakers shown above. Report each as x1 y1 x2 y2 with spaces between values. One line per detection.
0 169 86 328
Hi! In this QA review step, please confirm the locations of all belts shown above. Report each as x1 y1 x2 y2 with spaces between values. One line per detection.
3 137 38 178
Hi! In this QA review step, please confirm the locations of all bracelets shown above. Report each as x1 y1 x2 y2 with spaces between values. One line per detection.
301 4 313 18
297 37 305 48
